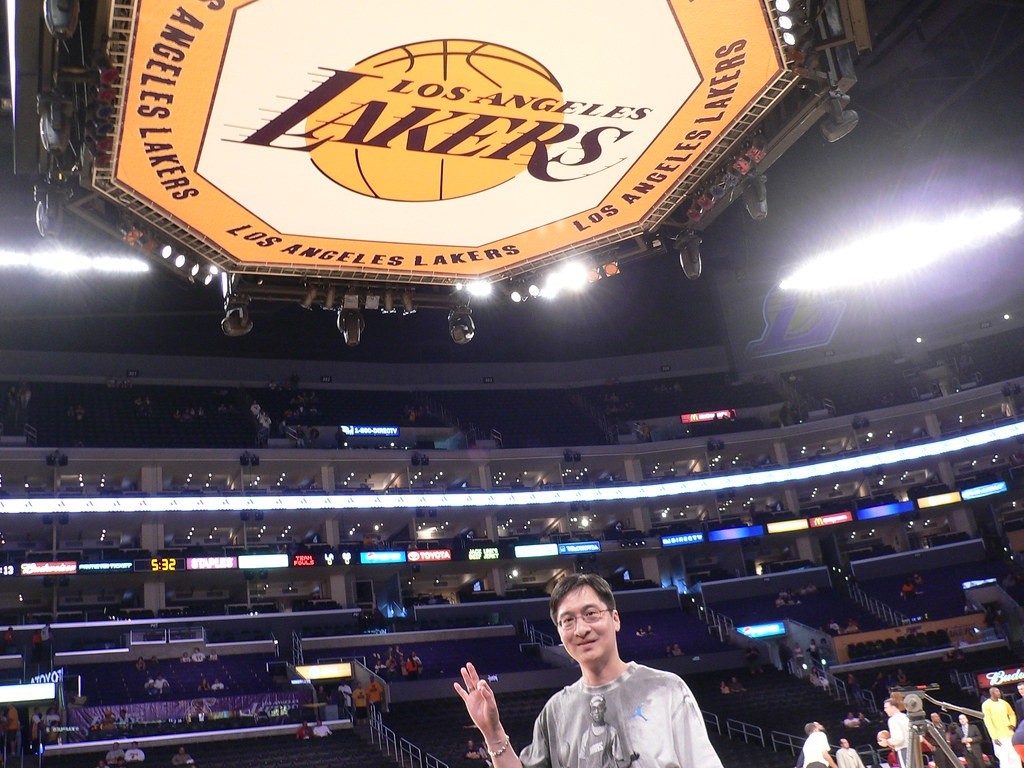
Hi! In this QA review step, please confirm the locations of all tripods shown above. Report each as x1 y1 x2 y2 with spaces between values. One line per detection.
891 691 984 768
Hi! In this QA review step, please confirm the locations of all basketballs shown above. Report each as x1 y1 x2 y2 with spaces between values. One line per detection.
877 730 890 742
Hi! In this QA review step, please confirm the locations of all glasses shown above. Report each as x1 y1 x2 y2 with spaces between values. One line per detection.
556 607 614 630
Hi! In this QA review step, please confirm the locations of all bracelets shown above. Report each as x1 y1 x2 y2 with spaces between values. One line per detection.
488 735 509 756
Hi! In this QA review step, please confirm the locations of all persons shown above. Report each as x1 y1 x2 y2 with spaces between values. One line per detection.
720 675 748 696
453 570 728 768
844 666 912 719
981 681 1024 768
463 737 494 768
929 711 987 768
836 739 866 768
0 702 63 768
664 640 687 658
95 647 270 768
776 578 817 611
635 623 658 638
749 499 776 525
880 698 918 768
793 721 839 768
1001 572 1024 595
844 710 869 728
985 606 1004 635
962 599 977 613
295 643 424 740
2 621 51 663
777 617 859 694
901 573 923 600
635 421 652 442
6 375 432 435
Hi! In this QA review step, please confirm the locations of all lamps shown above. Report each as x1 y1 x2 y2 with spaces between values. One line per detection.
80 49 119 169
119 221 212 286
671 228 704 281
43 0 79 40
220 271 252 336
815 92 858 143
33 176 75 239
447 290 475 346
511 251 620 302
35 88 76 153
302 283 415 347
775 0 818 78
686 136 770 222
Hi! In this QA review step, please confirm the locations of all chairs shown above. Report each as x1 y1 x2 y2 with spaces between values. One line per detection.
0 328 1024 768
